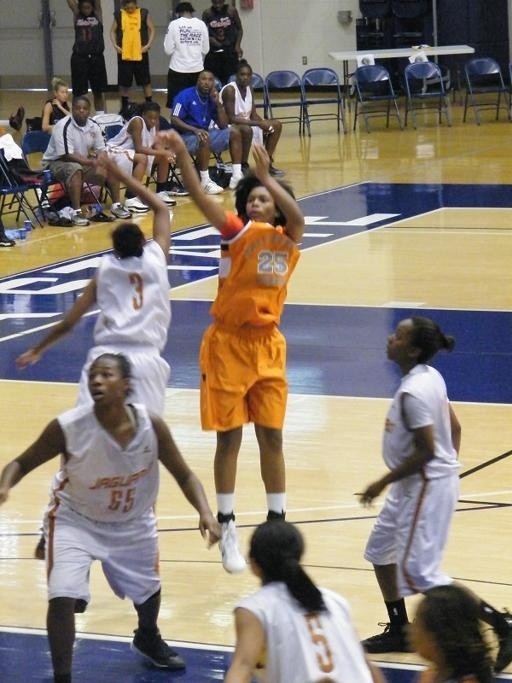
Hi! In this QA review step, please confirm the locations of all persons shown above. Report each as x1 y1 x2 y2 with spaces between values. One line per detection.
110 0 156 116
410 580 498 682
162 1 211 109
152 128 306 536
354 316 461 656
201 2 243 87
223 515 389 682
68 1 108 116
0 353 221 683
11 148 172 559
1 60 283 247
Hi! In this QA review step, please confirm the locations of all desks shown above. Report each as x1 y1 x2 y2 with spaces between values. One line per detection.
329 44 476 85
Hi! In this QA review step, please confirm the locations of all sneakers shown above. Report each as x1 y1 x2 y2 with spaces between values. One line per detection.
130 629 185 669
71 166 284 225
361 622 417 652
9 103 24 130
0 237 14 247
219 519 246 572
495 612 512 672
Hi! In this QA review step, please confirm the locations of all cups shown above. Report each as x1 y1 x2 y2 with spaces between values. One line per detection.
18 228 26 239
42 170 52 183
23 221 32 233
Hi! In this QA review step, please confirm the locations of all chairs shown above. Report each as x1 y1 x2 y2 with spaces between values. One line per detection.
23 130 60 223
404 62 450 130
301 68 347 136
454 56 512 125
63 149 225 209
265 70 310 137
352 65 403 134
229 73 267 117
0 146 46 229
